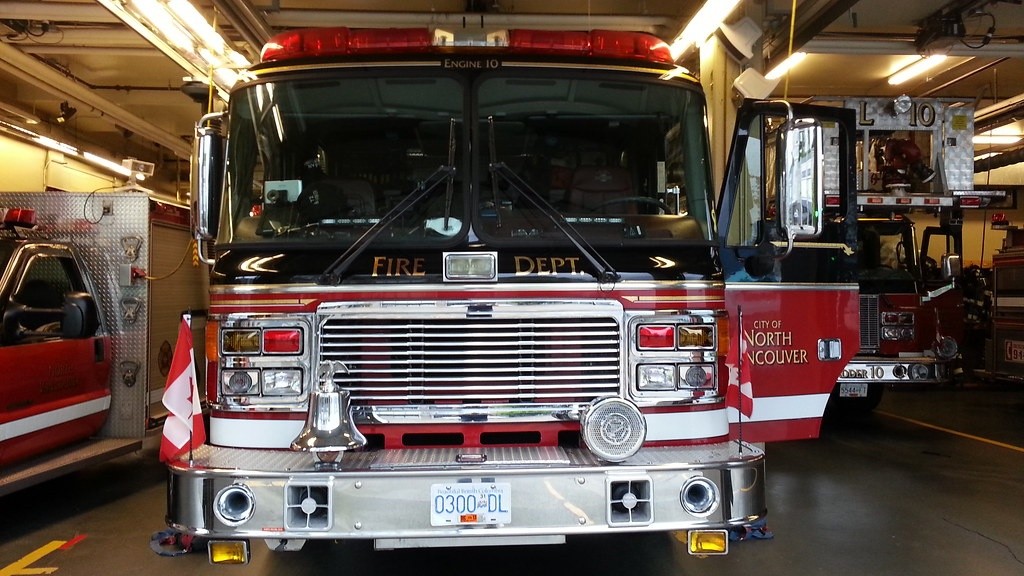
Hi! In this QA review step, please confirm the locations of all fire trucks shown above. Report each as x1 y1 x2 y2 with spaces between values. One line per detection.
766 95 1006 412
165 0 862 561
1 159 205 498
983 212 1024 383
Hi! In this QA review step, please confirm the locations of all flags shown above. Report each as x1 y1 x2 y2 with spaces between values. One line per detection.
725 313 753 419
158 313 206 464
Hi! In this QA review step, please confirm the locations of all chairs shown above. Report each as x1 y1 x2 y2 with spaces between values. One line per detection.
570 166 638 217
19 280 62 331
297 177 375 219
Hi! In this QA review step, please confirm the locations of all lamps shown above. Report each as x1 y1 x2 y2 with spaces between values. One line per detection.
110 158 157 196
55 101 77 124
